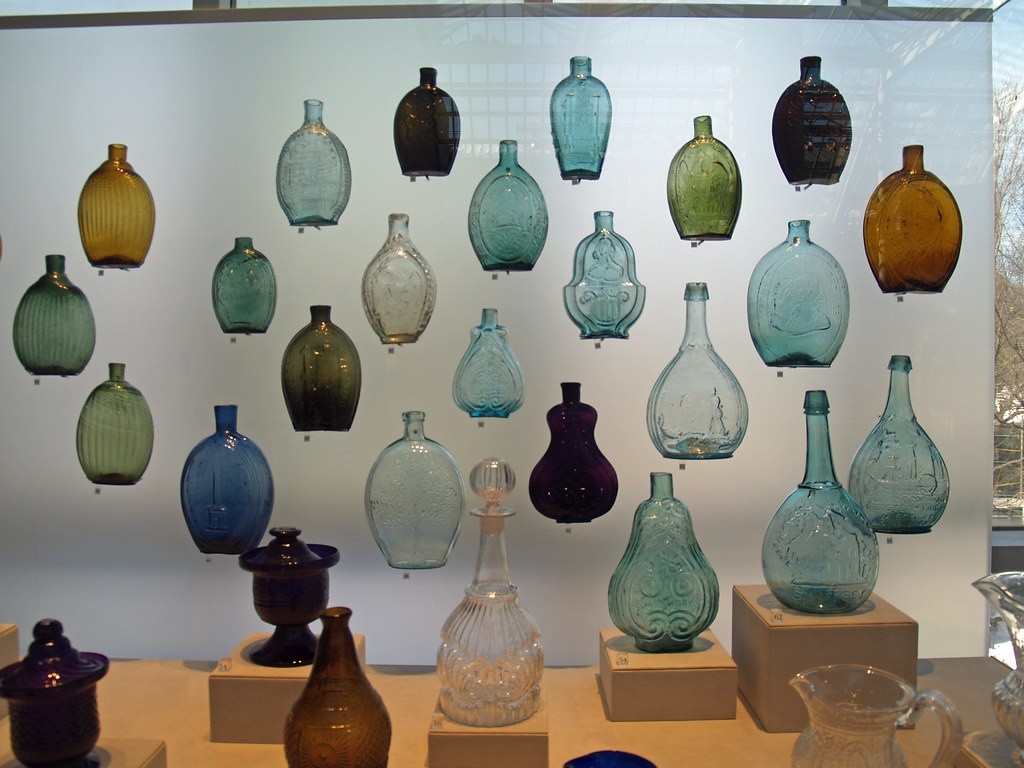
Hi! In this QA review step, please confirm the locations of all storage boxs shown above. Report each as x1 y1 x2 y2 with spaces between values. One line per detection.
1 47 1024 768
